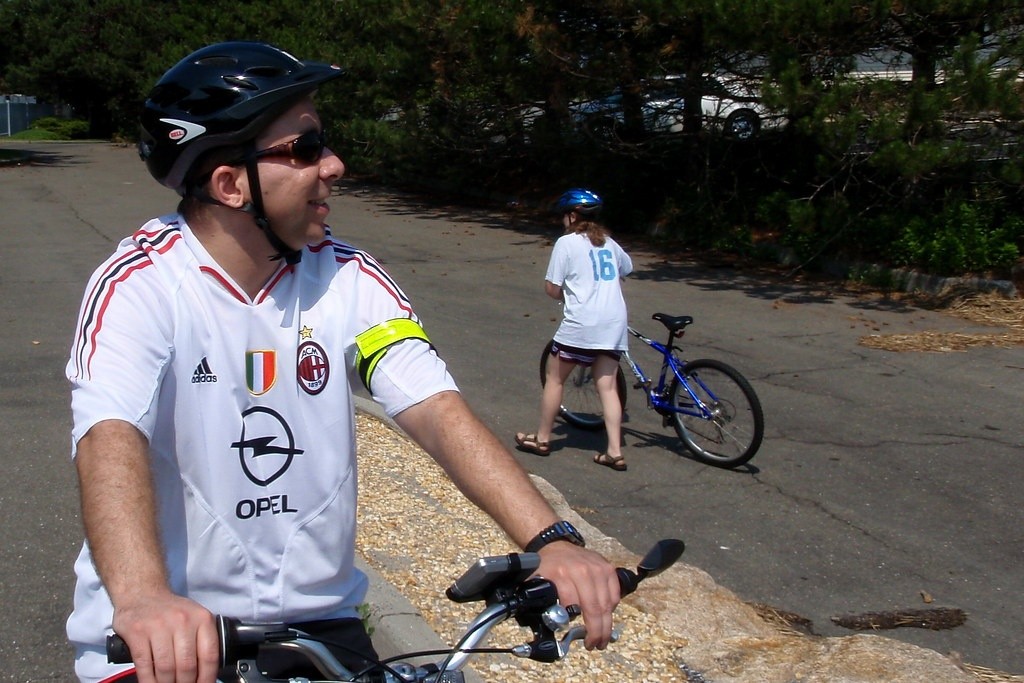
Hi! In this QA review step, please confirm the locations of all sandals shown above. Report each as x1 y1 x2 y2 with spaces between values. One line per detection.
514 432 550 456
594 451 627 471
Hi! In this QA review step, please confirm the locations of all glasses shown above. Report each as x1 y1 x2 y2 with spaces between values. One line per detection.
195 128 329 189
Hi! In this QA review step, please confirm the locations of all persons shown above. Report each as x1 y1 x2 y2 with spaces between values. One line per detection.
514 187 635 470
65 43 623 683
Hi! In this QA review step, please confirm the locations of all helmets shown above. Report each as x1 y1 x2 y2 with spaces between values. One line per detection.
139 41 350 198
556 187 604 215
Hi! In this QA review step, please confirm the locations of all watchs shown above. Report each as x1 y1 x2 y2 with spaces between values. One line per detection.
522 520 586 553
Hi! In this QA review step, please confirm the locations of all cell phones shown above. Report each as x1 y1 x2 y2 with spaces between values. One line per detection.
452 552 541 597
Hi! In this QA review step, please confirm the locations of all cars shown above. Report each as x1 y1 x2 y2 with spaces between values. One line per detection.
569 74 785 141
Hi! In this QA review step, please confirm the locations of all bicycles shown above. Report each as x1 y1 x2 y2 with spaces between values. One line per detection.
540 312 765 467
105 535 686 683
